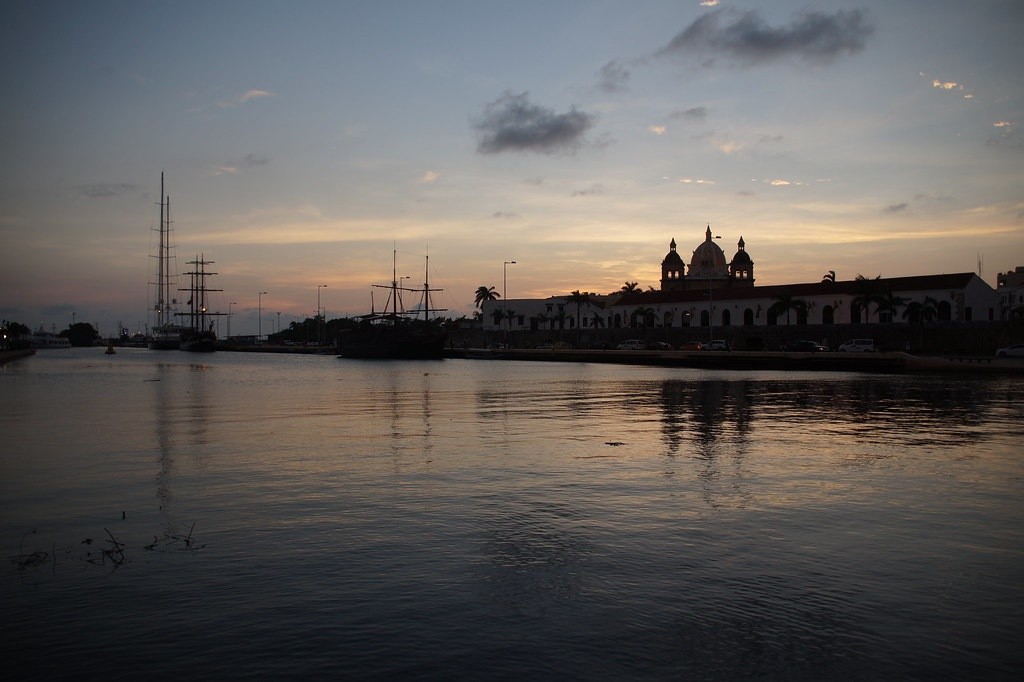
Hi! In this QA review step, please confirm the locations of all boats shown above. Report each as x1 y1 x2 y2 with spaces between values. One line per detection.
21 334 73 348
104 343 116 354
120 334 149 347
330 239 467 358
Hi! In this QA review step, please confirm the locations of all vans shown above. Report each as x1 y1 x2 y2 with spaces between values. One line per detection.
700 339 733 352
838 339 875 352
617 339 647 349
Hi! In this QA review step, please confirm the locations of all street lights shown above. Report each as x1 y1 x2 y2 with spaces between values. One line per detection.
709 235 722 350
317 284 328 346
258 291 269 345
227 302 237 342
503 261 517 346
399 276 410 321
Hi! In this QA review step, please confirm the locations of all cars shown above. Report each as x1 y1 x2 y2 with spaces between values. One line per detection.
795 340 830 352
653 342 672 350
995 344 1024 359
679 340 702 350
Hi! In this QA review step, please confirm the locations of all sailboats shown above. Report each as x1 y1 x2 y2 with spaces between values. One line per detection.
172 251 226 352
143 165 188 349
